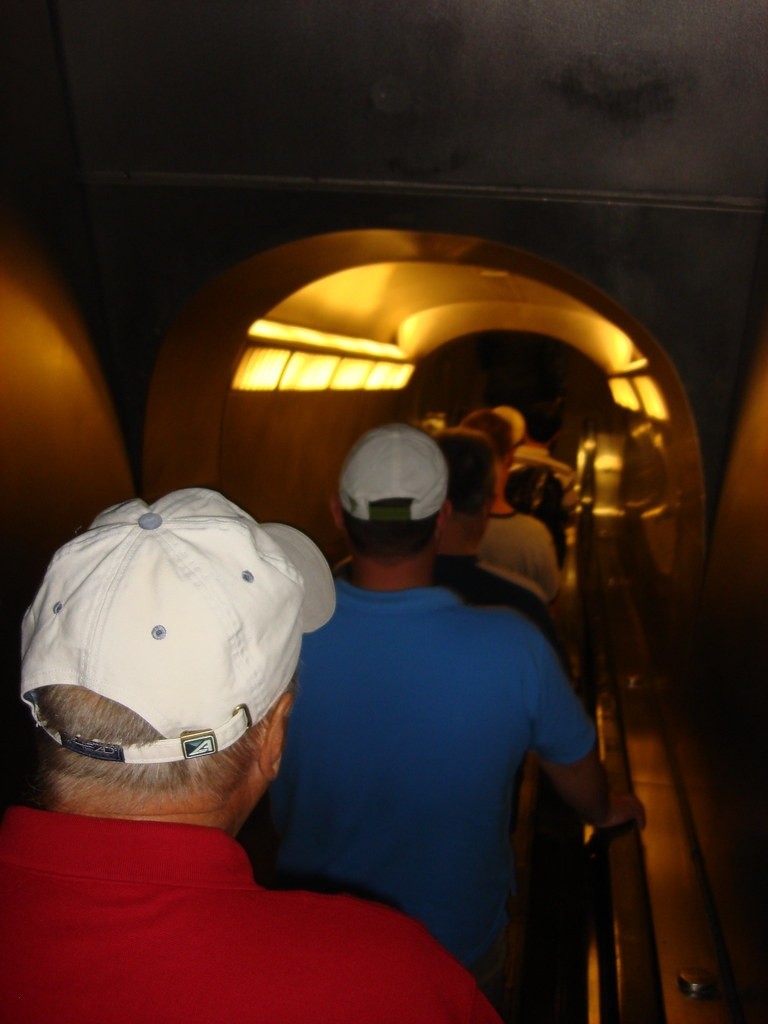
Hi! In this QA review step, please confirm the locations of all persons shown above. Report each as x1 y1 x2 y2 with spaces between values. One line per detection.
270 425 647 1006
0 407 647 1024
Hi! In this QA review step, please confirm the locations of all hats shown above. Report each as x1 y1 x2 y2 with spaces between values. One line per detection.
492 406 547 472
19 488 336 764
338 423 449 521
